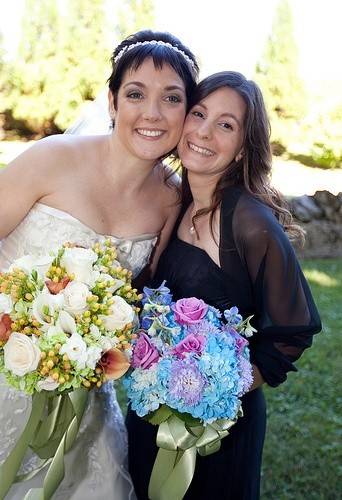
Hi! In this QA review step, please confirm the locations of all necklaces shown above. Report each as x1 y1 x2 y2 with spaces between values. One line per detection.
190 226 197 234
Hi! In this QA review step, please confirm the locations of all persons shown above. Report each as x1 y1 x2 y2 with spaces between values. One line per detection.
1 28 200 500
125 71 323 500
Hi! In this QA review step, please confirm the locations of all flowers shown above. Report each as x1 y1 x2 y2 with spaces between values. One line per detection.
0 238 258 500
